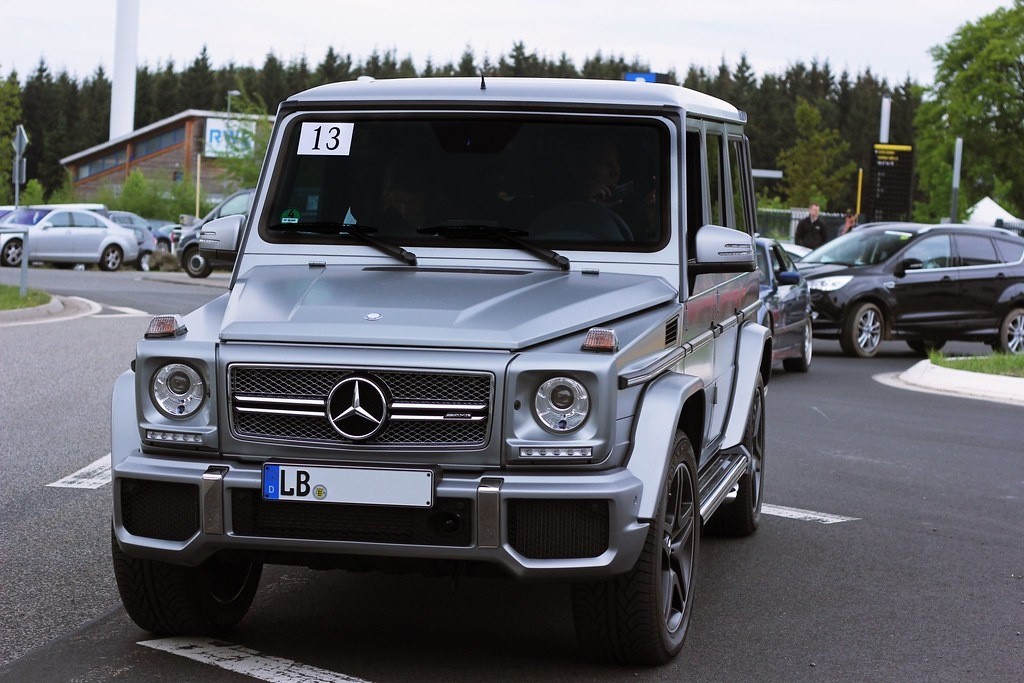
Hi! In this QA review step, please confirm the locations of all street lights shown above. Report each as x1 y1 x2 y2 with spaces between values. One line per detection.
222 89 241 200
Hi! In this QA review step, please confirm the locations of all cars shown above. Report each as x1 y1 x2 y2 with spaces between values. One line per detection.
767 241 832 267
0 202 181 273
752 238 813 374
0 204 138 272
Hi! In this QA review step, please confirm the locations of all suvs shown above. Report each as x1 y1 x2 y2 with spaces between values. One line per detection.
111 73 774 670
792 221 1024 360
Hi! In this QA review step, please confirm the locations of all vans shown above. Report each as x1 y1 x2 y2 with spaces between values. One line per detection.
176 186 257 278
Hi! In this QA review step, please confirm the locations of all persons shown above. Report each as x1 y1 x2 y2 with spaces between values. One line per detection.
837 207 861 236
355 132 659 244
794 202 828 250
